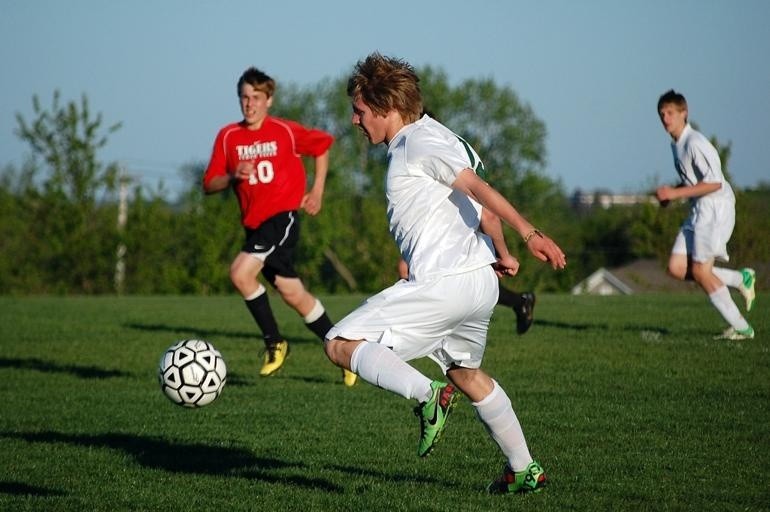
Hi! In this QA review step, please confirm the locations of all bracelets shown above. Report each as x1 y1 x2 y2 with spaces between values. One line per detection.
524 227 541 244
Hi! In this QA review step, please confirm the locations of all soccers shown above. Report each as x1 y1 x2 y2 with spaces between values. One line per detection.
158 337 229 411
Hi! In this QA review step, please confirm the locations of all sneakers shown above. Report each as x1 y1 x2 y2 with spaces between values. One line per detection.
488 459 547 496
736 268 756 315
342 368 357 386
513 293 536 335
711 325 754 341
417 382 461 458
260 340 291 377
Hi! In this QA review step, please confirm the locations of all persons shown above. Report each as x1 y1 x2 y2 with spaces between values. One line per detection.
415 104 537 336
321 49 570 496
201 67 358 388
655 89 759 342
